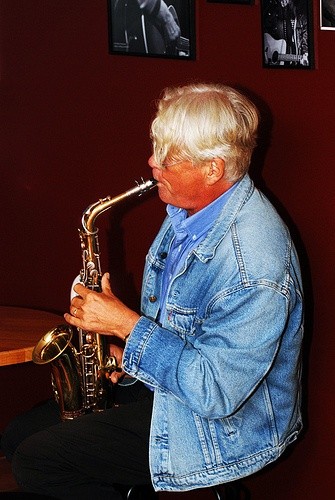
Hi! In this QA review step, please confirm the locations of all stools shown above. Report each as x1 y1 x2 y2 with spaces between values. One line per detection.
125 482 250 500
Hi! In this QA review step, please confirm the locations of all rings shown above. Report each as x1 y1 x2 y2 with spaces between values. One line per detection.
73 308 78 317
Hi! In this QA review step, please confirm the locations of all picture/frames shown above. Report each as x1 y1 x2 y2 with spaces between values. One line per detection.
319 0 335 31
107 0 197 62
260 0 316 70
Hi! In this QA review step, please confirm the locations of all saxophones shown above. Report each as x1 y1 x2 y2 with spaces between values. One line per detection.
30 179 157 424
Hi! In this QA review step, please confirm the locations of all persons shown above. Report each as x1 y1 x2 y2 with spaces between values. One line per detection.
0 83 306 500
137 0 181 55
265 0 308 66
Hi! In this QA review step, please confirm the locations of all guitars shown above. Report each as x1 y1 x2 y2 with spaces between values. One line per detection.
263 32 308 65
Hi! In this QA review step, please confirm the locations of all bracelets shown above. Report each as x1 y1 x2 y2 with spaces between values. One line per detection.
123 333 130 342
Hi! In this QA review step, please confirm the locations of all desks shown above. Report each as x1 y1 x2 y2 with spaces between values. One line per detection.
0 305 72 367
